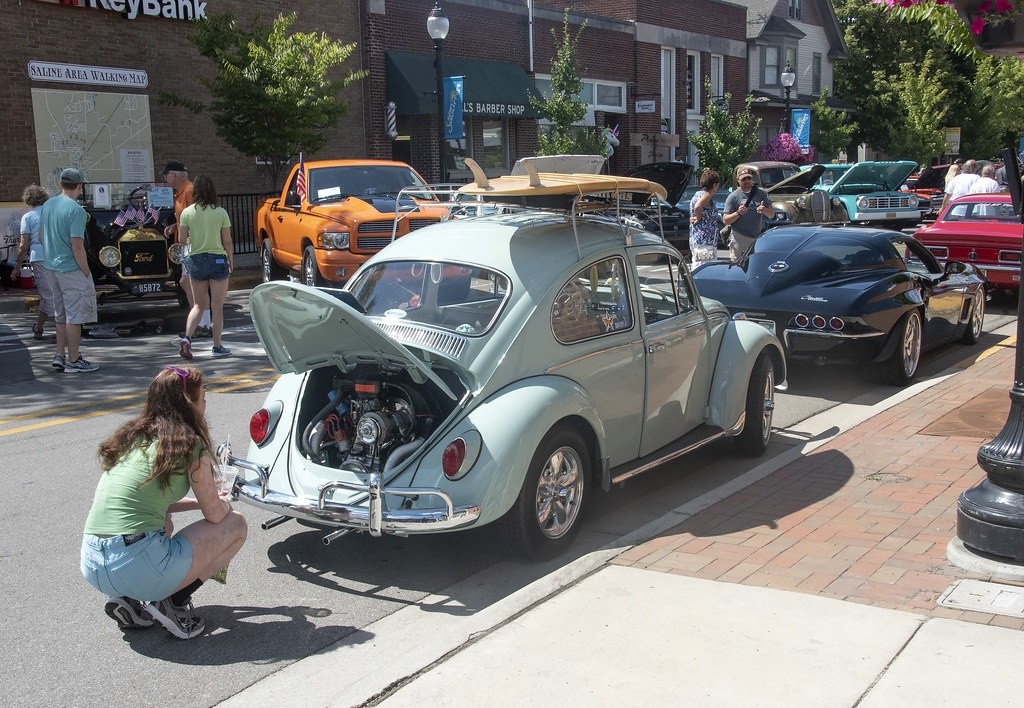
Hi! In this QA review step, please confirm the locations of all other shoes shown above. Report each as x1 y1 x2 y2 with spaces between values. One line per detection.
32 323 44 337
211 345 231 357
179 324 213 338
47 335 56 343
179 336 194 360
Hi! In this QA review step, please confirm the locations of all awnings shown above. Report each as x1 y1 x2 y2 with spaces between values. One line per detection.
384 50 548 120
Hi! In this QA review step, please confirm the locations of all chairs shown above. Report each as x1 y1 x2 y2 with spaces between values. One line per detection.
850 250 882 267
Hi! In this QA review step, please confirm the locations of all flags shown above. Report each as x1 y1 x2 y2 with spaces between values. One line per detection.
148 203 155 213
114 210 128 226
135 206 144 222
152 210 158 225
124 205 137 220
296 154 307 205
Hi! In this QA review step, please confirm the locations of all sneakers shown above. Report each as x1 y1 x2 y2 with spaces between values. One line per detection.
104 596 154 629
64 356 101 372
141 595 206 639
51 353 67 369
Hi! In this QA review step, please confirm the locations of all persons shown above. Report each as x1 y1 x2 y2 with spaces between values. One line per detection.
11 184 55 345
158 161 213 339
38 168 100 374
79 364 249 640
176 173 231 359
935 157 1009 223
722 166 775 270
689 170 721 265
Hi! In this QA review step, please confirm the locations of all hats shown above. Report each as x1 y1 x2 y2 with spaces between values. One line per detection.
158 161 187 175
737 166 753 180
60 168 89 185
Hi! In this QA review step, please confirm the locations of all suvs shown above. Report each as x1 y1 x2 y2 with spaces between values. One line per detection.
76 179 191 309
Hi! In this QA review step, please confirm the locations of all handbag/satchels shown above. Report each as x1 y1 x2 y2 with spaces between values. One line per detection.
720 224 732 246
693 246 715 262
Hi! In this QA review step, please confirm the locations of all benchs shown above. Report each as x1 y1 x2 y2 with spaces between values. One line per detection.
442 306 492 326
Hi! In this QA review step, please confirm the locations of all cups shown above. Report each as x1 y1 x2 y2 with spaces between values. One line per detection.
212 465 238 499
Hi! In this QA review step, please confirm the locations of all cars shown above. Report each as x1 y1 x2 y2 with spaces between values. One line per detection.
215 205 784 564
254 152 1023 310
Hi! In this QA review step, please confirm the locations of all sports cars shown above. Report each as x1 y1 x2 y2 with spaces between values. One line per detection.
689 224 991 390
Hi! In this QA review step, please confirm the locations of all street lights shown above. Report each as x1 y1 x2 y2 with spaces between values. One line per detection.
781 60 796 134
426 2 451 186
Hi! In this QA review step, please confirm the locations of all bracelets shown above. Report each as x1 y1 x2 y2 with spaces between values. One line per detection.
14 262 22 268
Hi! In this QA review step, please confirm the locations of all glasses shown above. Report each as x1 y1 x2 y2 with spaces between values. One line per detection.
742 176 751 181
162 366 190 393
